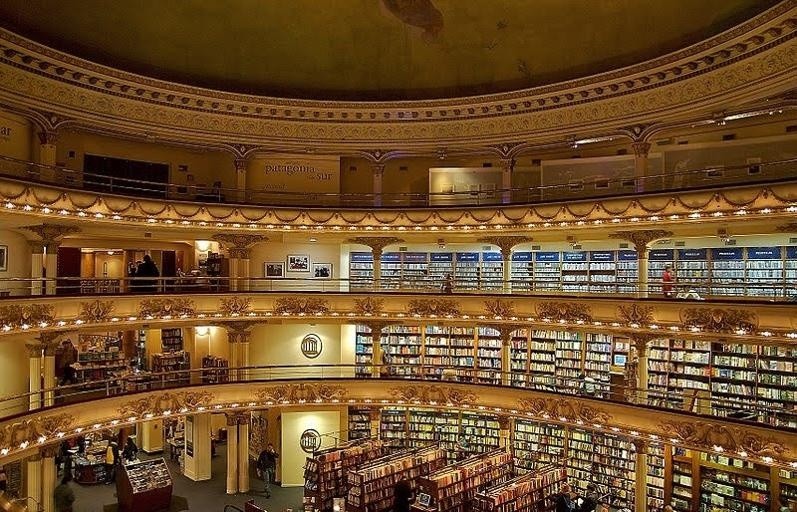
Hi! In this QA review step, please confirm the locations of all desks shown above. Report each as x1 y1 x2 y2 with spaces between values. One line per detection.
165 437 185 462
70 362 127 390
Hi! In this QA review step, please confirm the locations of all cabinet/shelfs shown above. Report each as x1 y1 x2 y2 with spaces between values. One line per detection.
351 257 796 296
202 356 229 382
300 406 636 512
152 328 191 384
642 325 796 433
509 325 636 403
646 439 797 512
353 325 502 384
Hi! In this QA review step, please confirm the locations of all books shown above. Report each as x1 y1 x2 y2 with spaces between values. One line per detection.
69 328 229 394
126 461 173 494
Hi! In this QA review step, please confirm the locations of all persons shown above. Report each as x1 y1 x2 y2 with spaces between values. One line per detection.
54 477 75 510
1 469 10 491
137 254 160 295
59 339 77 387
255 443 279 500
104 438 139 485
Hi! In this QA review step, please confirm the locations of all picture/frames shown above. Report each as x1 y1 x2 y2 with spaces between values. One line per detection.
263 253 332 281
